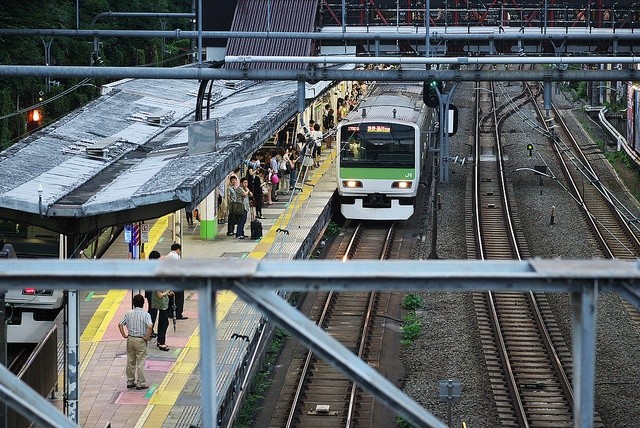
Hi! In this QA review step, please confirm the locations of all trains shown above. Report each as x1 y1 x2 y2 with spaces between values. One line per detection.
334 64 448 220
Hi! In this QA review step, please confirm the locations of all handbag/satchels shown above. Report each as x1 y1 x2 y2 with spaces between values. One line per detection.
272 174 278 183
233 186 244 215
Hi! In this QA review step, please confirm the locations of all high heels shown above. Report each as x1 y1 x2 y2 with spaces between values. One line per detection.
159 345 169 351
157 343 168 347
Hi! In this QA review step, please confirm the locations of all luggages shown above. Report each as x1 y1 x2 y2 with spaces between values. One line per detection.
251 205 262 239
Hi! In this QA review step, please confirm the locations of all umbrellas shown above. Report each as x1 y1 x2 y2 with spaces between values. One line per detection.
171 291 177 334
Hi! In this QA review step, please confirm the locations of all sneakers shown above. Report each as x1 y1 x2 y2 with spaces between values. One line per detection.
237 235 243 239
228 232 233 236
243 235 248 237
233 231 236 234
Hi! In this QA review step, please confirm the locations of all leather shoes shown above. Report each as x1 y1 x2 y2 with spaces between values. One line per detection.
128 385 136 388
177 315 188 319
137 385 149 389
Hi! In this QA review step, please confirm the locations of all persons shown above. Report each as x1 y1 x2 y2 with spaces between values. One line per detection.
228 177 240 236
166 244 189 320
186 206 195 227
247 63 384 218
119 294 153 392
236 177 253 239
148 250 174 354
229 169 241 187
